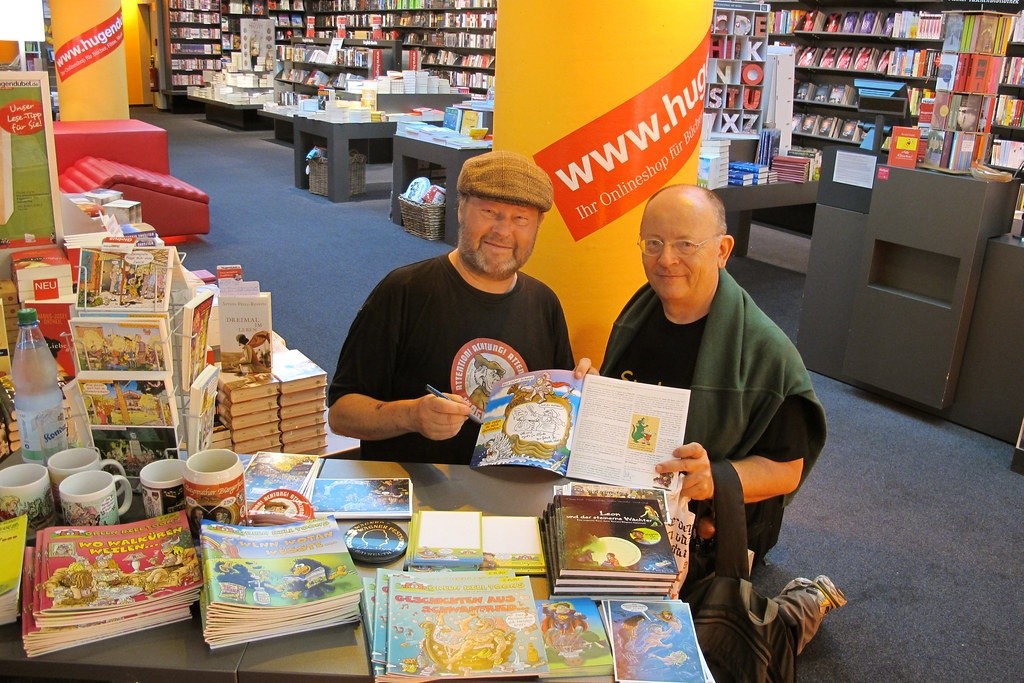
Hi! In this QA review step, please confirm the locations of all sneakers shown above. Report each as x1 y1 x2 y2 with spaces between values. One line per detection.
780 575 848 615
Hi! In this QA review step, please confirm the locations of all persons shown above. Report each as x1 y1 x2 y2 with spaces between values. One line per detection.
322 151 601 468
597 184 848 654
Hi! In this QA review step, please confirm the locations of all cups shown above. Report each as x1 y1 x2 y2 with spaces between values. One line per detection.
140 459 187 518
184 448 248 539
0 463 58 540
59 470 133 525
48 447 127 519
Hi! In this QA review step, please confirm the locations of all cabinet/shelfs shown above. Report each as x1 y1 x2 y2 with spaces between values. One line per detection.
712 140 819 258
767 31 1024 176
163 1 497 246
704 1 770 139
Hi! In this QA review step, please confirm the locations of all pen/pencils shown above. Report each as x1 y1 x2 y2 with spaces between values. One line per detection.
424 384 484 424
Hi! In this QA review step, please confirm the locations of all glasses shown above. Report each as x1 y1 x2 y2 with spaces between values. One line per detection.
638 234 721 257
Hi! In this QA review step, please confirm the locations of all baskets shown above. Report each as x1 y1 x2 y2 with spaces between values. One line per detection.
308 154 366 196
397 195 445 240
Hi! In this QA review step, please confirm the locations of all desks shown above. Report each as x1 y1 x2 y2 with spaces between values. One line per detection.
0 451 602 683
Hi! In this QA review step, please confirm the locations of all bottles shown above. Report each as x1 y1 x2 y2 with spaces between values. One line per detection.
12 308 67 466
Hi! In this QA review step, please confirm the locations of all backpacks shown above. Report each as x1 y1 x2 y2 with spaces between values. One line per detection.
682 460 799 683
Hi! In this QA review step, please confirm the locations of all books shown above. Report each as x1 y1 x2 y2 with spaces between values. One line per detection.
22 0 498 141
696 9 1024 218
469 370 690 495
0 188 717 683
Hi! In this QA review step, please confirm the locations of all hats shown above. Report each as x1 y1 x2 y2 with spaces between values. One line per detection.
457 152 553 214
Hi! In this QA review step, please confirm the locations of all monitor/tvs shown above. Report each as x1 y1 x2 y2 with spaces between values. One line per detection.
854 79 912 127
